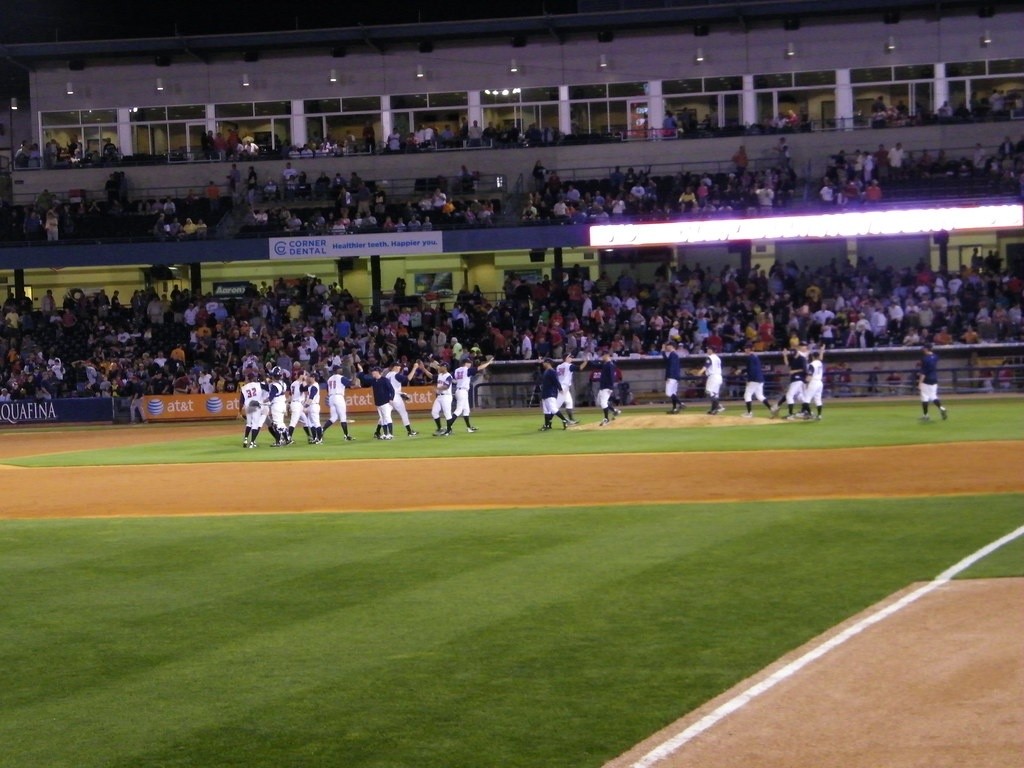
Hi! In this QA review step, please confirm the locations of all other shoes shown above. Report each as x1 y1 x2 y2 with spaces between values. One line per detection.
242 436 322 448
920 415 930 421
600 418 609 426
742 412 752 417
680 405 686 410
667 409 678 414
707 407 725 414
344 435 356 441
942 409 946 421
612 409 621 421
373 427 479 440
768 408 822 420
540 418 581 431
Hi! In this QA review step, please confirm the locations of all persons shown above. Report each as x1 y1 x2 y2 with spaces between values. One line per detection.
264 367 293 446
736 342 772 418
535 357 569 432
771 348 813 420
452 357 494 433
238 376 262 448
0 87 1024 402
322 365 356 440
585 350 621 426
374 362 420 439
698 346 725 415
557 353 588 425
305 373 322 445
358 362 395 440
795 344 825 420
661 342 686 414
253 375 277 446
917 342 947 421
287 370 313 444
416 359 454 436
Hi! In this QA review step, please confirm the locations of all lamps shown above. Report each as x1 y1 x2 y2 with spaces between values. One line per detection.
242 74 250 87
510 59 518 73
600 55 607 68
329 68 337 83
10 97 18 110
696 47 706 63
66 82 74 95
982 29 991 44
887 36 897 52
156 77 163 91
415 64 425 78
786 42 796 57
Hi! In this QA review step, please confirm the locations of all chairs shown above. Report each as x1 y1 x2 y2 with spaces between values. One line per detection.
239 199 500 234
562 172 727 199
0 196 234 242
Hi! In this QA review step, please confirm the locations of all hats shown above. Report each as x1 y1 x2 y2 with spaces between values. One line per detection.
371 367 381 373
333 365 342 371
438 362 449 367
